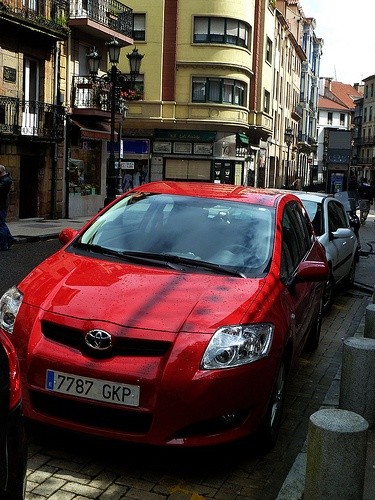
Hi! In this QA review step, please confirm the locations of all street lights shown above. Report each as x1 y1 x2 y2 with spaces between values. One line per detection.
283 125 294 190
106 40 144 204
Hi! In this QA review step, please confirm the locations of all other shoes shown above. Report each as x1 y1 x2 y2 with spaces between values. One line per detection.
0 246 9 251
361 218 365 225
8 239 17 247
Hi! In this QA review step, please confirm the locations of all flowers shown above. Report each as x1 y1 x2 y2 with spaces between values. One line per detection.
89 79 113 105
119 86 144 102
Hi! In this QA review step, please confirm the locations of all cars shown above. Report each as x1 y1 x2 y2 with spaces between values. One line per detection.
236 188 361 313
1 183 328 452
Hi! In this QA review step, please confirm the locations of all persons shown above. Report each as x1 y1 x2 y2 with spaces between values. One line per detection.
292 177 303 190
356 178 373 225
0 164 16 250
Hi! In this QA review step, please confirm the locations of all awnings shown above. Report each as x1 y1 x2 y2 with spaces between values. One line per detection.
71 119 117 141
